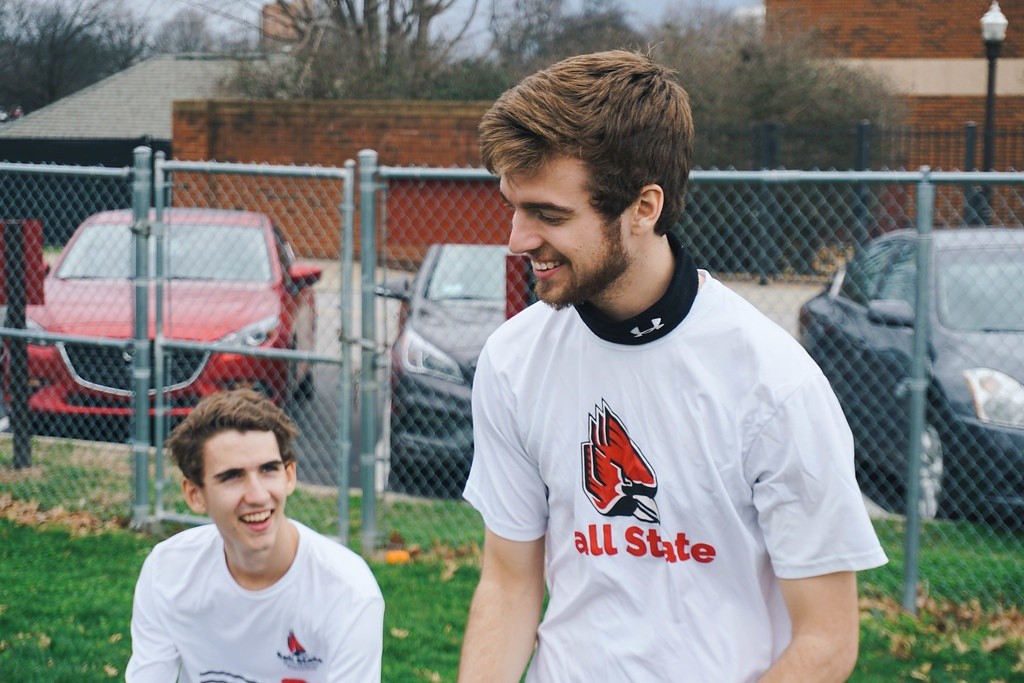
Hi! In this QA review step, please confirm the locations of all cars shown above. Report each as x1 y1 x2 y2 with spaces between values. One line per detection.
798 227 1024 523
373 241 539 498
5 209 323 442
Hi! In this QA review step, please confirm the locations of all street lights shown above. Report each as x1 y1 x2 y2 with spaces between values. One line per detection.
979 0 1008 228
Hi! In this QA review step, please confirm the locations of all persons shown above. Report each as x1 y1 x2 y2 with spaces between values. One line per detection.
124 387 386 683
456 40 890 682
